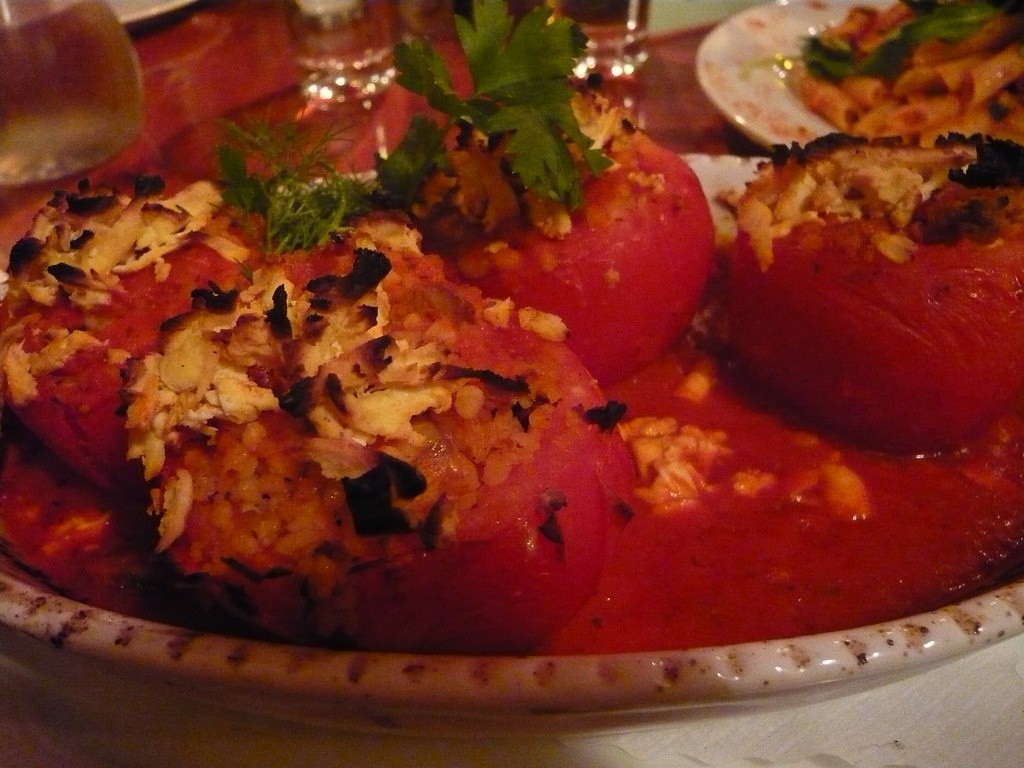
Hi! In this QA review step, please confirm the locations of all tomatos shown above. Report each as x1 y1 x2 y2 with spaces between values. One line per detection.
0 122 1024 650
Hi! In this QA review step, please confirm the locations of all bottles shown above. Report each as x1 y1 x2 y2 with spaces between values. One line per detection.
1 1 148 184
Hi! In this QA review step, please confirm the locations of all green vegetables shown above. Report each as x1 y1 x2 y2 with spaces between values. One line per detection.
799 0 1024 80
211 0 614 278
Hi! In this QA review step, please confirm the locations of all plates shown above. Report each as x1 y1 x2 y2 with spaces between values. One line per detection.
111 1 195 28
697 1 897 156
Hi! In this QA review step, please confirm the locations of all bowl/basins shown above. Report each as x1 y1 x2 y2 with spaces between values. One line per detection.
0 156 1024 734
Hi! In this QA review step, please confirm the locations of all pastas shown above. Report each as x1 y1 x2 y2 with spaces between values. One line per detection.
786 0 1024 147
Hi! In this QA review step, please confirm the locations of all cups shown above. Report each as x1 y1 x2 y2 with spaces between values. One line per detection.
283 0 397 103
548 0 647 81
